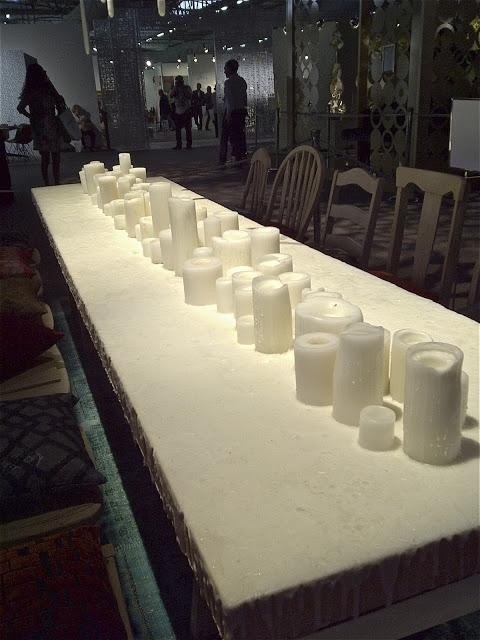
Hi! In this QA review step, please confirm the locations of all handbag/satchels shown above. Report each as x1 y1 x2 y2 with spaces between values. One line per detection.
54 96 82 143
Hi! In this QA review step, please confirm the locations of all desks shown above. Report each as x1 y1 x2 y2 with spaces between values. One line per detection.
30 176 478 638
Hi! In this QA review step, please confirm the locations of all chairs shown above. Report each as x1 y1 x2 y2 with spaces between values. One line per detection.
5 124 36 163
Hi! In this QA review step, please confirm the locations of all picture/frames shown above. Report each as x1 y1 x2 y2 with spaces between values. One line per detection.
381 44 396 73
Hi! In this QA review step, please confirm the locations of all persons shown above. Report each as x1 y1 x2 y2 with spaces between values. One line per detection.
15 63 66 186
71 104 99 153
210 84 221 138
203 86 213 131
329 66 345 95
213 59 248 166
166 103 177 131
157 88 171 130
170 75 193 151
193 83 204 132
